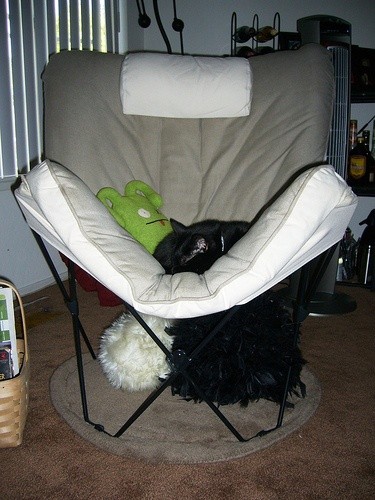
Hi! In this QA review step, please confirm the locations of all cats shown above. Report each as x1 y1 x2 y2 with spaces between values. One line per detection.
152 218 251 274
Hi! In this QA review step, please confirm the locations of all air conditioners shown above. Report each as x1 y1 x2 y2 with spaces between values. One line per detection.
295 14 352 316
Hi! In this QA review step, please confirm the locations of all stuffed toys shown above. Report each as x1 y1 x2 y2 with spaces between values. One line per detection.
96 180 174 255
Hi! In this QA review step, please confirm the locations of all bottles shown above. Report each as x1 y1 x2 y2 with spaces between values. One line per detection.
363 130 375 181
371 120 375 159
350 119 357 150
349 138 371 181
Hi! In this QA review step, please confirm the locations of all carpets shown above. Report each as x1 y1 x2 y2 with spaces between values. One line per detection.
49 347 322 463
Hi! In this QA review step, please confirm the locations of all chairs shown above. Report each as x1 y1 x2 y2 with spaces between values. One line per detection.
12 43 359 441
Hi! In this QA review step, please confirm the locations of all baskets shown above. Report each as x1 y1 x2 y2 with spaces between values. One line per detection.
0 280 29 449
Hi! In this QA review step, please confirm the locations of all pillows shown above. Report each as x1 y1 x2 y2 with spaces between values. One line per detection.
120 52 253 118
